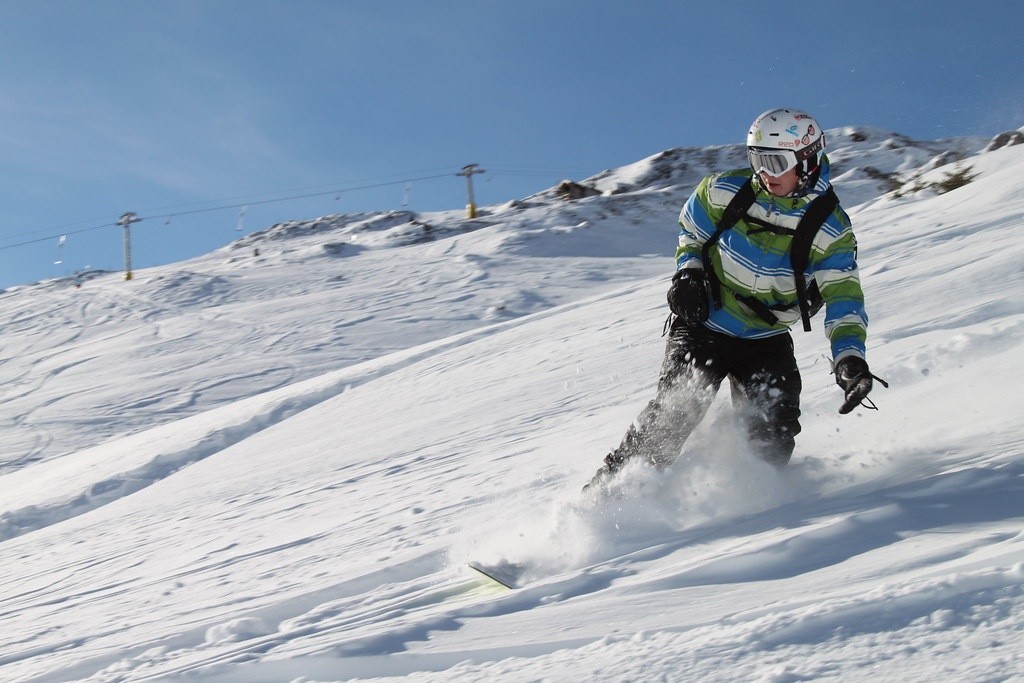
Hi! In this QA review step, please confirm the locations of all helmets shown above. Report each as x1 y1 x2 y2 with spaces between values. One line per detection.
747 108 826 181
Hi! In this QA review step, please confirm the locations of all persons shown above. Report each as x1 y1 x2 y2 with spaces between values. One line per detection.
580 106 875 508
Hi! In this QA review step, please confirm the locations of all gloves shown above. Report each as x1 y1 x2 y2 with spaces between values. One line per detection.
836 355 873 410
666 269 710 327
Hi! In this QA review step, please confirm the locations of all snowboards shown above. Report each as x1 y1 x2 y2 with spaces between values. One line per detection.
467 558 546 593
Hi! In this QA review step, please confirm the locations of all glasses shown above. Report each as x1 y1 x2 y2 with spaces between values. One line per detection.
748 134 825 177
